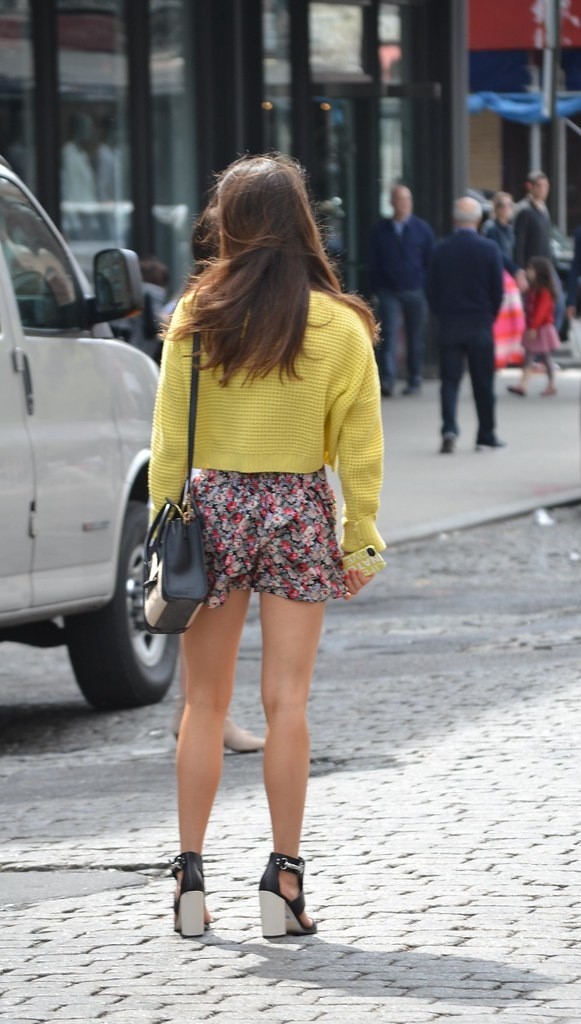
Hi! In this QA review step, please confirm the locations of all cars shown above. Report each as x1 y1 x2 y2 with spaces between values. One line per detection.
0 147 173 709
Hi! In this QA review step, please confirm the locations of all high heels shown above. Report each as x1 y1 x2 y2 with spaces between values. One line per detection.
171 703 266 752
257 853 316 936
165 851 208 936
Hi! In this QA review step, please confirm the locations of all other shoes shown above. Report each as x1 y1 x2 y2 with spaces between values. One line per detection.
403 383 423 396
381 380 393 399
507 386 527 394
541 386 557 395
438 431 454 451
474 442 507 454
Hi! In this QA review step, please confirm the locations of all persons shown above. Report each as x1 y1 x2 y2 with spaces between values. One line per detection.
366 171 581 453
148 156 385 939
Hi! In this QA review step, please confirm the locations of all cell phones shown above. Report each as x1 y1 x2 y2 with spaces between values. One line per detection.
342 545 386 578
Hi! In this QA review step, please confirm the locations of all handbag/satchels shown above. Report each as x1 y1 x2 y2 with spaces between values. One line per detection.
140 497 208 636
